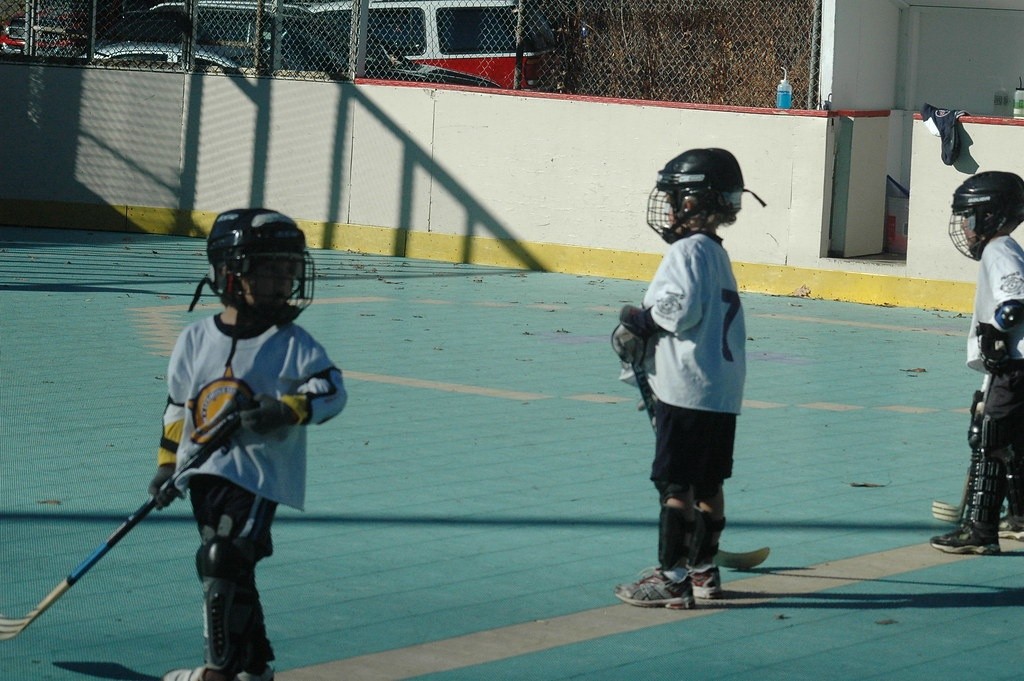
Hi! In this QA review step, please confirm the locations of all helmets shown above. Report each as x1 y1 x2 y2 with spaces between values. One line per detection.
208 207 305 299
952 171 1024 237
657 148 743 224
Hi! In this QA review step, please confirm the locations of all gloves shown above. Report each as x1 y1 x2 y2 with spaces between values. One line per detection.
148 463 178 510
610 323 646 368
977 323 1010 375
240 393 300 436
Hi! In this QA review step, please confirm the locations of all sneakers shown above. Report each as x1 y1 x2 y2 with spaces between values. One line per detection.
999 514 1024 540
614 566 695 610
930 518 1000 554
684 564 722 598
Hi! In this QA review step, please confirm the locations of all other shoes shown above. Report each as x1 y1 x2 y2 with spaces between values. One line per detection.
236 664 275 681
161 666 239 681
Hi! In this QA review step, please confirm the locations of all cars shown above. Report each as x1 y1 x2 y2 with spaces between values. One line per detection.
0 0 571 94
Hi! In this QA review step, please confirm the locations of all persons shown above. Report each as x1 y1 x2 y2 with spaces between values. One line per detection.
930 170 1024 554
149 209 349 680
614 147 745 609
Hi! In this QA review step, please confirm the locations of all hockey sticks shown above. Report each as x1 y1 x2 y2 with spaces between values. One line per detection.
0 413 242 640
618 335 772 570
930 336 1004 522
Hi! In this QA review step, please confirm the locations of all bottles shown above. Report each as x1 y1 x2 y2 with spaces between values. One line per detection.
776 79 792 109
1013 87 1024 119
